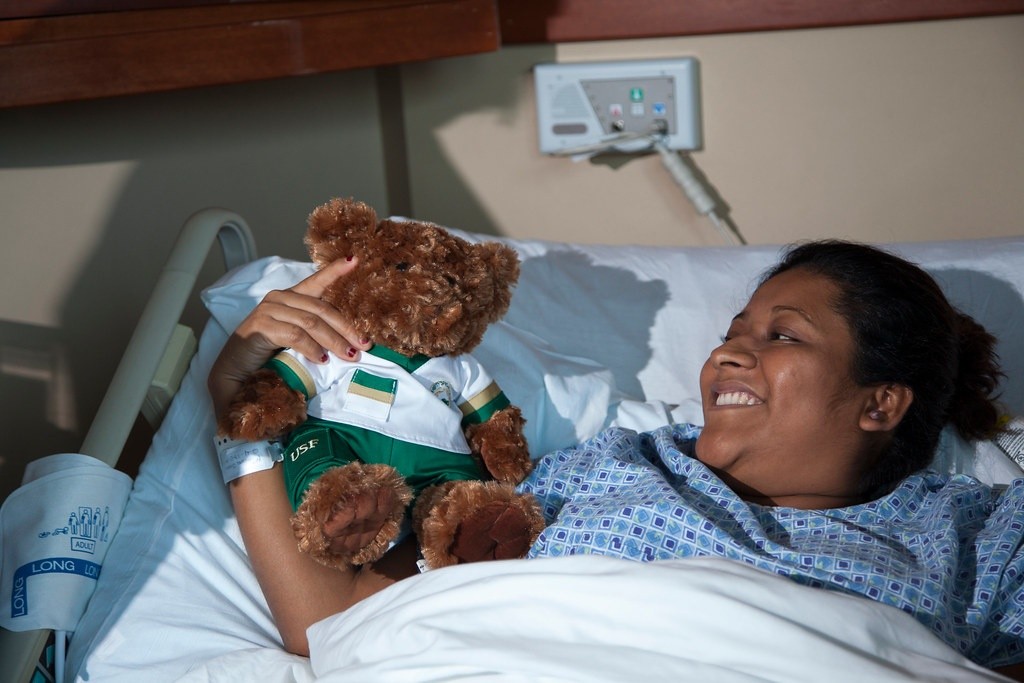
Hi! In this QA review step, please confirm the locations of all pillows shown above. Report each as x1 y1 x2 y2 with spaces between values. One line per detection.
208 217 1024 500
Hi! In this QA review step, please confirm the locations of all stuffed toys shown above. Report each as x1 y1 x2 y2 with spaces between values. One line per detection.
217 198 545 570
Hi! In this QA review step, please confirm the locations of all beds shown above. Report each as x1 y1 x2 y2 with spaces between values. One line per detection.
0 198 1024 683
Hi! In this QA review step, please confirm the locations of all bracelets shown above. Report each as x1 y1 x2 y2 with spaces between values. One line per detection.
212 430 286 485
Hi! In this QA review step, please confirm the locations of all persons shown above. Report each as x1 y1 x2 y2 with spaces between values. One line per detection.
208 238 1024 683
